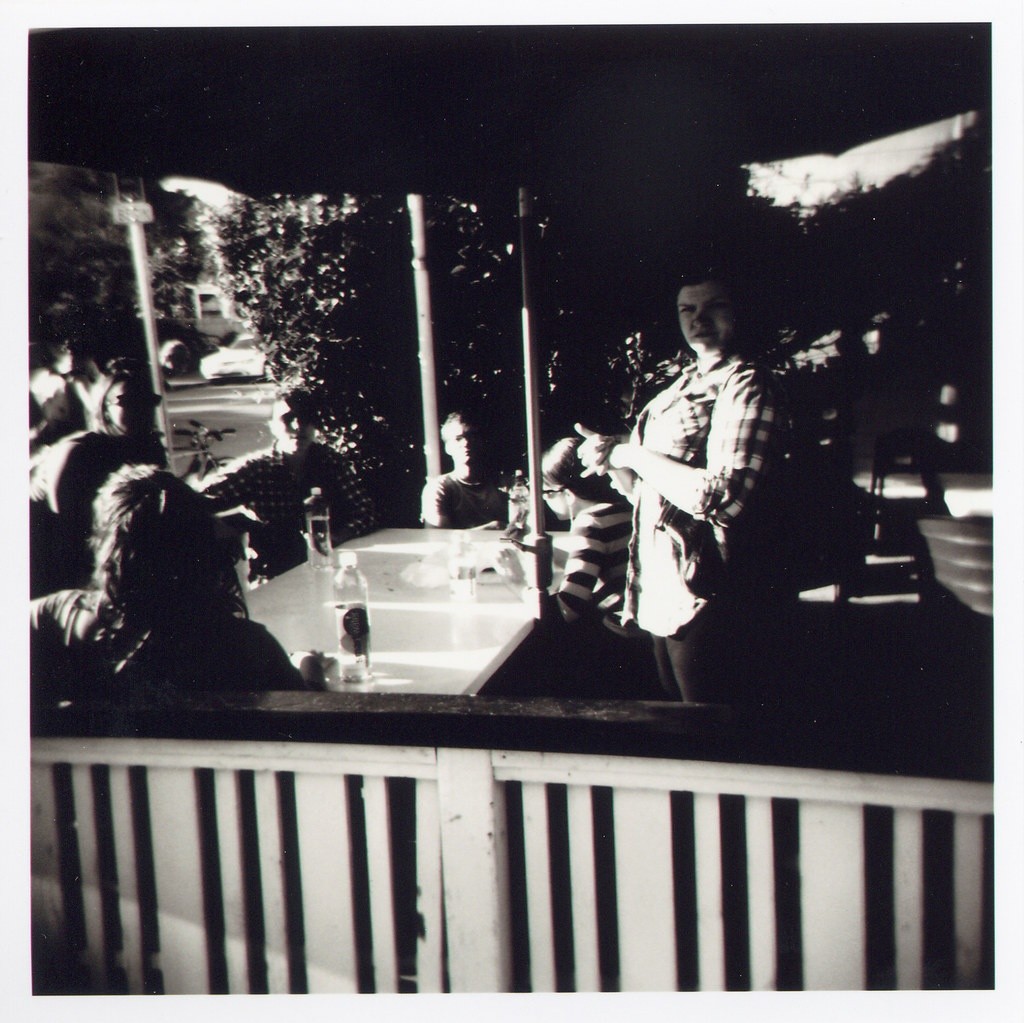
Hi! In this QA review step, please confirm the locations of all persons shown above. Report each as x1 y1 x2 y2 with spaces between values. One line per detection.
30 331 166 587
872 443 943 504
30 465 335 691
422 411 511 529
493 438 641 638
575 265 788 704
198 383 376 571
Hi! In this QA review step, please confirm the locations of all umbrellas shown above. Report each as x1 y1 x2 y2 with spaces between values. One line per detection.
29 22 992 599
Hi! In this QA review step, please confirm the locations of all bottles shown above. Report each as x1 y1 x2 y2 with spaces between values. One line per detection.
303 487 332 568
507 469 529 523
333 552 371 683
442 531 479 600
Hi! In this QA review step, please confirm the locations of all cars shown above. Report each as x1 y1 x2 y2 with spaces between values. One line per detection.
199 335 284 385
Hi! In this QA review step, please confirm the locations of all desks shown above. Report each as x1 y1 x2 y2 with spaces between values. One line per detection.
245 526 572 697
